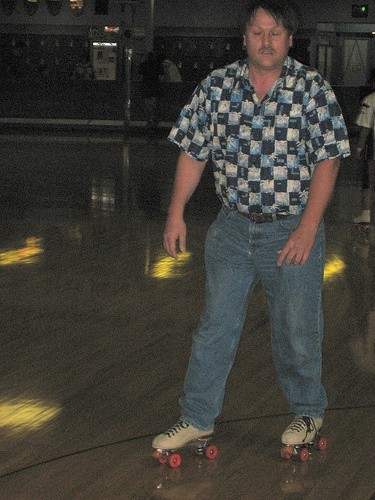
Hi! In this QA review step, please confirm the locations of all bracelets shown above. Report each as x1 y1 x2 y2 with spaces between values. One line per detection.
357 147 363 153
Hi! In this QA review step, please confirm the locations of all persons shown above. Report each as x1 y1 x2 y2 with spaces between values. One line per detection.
71 54 97 87
137 52 199 121
352 68 375 230
151 1 350 468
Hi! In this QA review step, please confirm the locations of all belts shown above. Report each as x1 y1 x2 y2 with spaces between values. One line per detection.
236 211 282 224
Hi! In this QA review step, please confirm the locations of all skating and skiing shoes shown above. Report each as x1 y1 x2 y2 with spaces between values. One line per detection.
354 210 371 231
280 411 328 463
151 418 218 469
